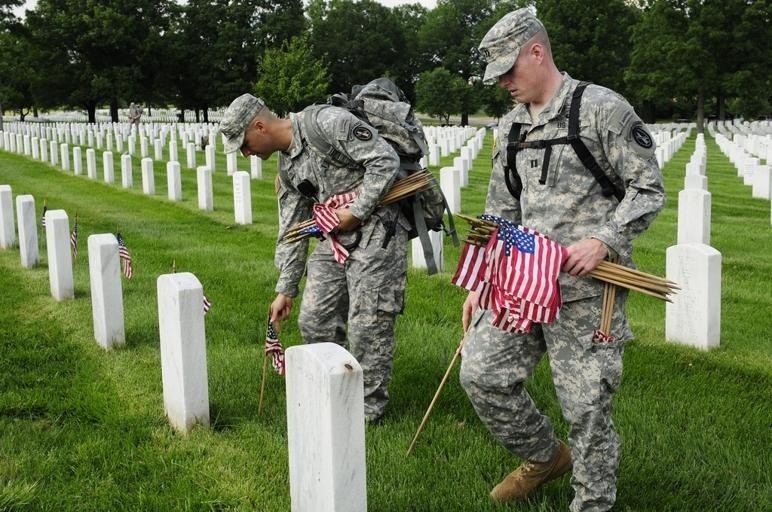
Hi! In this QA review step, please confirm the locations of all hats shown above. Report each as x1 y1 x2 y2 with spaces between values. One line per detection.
477 7 544 87
219 93 266 155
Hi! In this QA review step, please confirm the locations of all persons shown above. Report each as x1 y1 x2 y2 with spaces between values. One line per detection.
126 101 141 136
457 7 666 512
216 90 413 424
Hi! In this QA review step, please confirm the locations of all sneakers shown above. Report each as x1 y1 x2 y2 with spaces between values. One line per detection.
488 438 573 504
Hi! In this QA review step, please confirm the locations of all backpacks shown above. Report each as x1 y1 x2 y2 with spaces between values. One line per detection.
302 76 448 242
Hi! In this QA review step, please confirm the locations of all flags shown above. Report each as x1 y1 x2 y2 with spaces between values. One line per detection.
203 295 211 315
70 223 78 258
41 205 48 227
265 323 284 377
450 214 569 335
299 190 359 267
117 232 132 279
